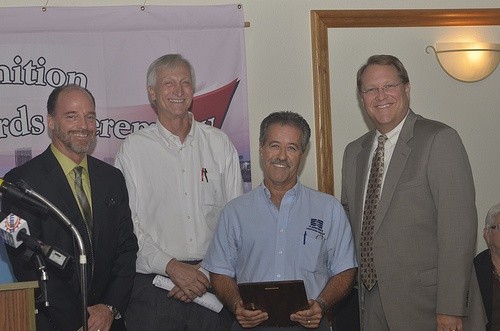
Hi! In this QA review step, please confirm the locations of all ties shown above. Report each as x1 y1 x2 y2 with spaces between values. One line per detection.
361 133 388 292
71 166 91 230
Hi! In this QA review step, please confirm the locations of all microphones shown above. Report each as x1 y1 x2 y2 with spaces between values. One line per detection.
0 209 74 270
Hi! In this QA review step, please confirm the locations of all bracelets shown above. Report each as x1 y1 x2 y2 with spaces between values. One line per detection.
317 300 329 313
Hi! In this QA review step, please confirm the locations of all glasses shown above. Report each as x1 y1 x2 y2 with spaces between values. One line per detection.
488 224 500 230
363 81 403 97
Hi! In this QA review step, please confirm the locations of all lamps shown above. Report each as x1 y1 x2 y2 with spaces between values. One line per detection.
425 41 500 83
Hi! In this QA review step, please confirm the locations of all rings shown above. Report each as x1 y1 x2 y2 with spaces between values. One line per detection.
308 321 310 325
97 330 100 331
451 330 455 331
184 295 188 299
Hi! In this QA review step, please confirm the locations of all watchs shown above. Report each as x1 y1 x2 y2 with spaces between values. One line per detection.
106 304 117 318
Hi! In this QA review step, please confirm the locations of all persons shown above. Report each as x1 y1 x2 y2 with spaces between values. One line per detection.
341 55 489 331
473 203 500 331
0 83 139 331
202 111 358 331
116 54 244 331
